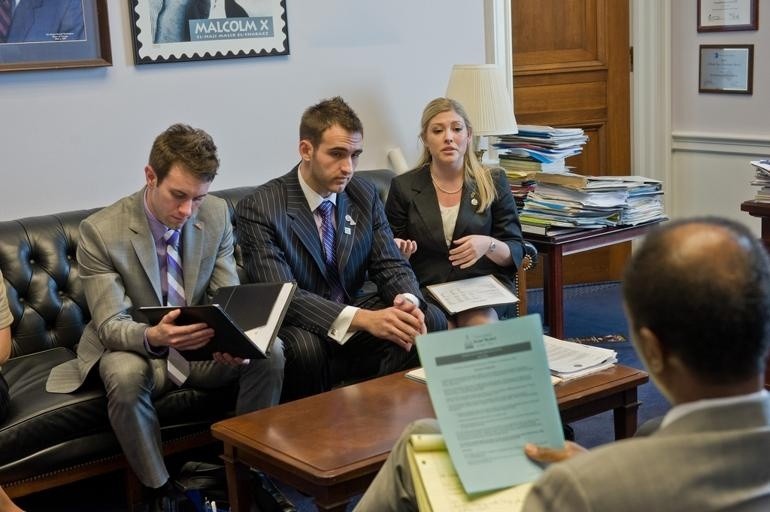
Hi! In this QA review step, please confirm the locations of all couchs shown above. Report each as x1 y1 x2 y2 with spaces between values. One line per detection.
0 166 541 512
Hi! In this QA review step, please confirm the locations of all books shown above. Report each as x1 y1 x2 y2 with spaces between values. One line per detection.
486 121 669 236
138 282 297 360
537 330 621 381
425 274 521 317
750 157 770 204
404 431 544 512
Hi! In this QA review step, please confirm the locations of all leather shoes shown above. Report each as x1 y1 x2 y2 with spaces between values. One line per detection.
144 460 305 512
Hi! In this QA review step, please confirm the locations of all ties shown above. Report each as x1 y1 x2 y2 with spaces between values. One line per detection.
164 228 190 391
317 200 343 316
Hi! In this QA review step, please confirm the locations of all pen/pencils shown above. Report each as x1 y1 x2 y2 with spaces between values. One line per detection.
204 496 217 512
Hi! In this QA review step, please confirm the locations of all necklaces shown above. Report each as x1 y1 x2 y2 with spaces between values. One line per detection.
430 174 464 194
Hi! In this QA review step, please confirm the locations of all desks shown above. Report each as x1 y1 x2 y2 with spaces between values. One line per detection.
523 208 667 342
739 199 769 247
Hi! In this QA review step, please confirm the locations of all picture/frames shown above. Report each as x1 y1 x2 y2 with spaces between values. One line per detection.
126 0 291 67
0 0 114 73
696 0 759 31
698 44 754 95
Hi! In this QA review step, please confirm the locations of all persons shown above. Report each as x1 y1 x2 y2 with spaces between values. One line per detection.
1 2 85 44
0 271 29 511
347 216 770 512
228 98 451 398
41 126 299 510
384 95 525 333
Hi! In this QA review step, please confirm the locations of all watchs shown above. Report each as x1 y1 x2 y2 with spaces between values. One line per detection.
487 237 497 256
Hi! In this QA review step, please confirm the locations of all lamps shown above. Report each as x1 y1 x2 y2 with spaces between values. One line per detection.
446 62 517 167
